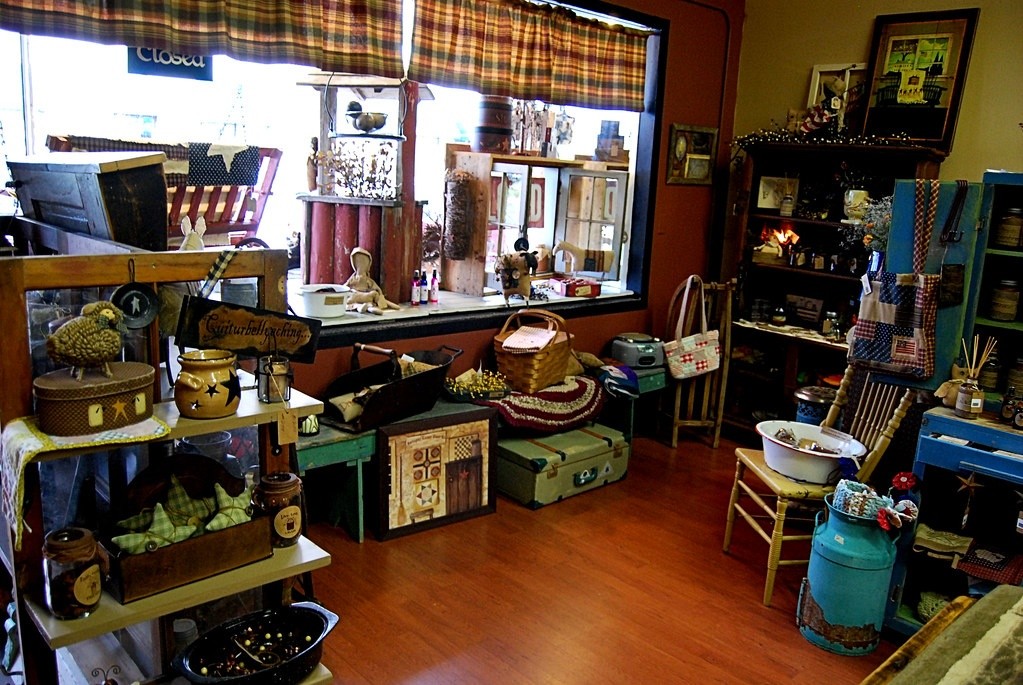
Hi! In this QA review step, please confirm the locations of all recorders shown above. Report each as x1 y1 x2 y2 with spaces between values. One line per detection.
612 332 666 368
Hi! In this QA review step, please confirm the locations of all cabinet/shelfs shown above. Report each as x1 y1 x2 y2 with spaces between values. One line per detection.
882 171 1023 634
721 137 948 438
0 213 334 685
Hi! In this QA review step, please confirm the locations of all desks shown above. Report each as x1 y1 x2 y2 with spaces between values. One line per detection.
295 423 377 543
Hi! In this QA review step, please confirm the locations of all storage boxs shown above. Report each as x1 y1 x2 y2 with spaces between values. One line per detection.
90 509 272 604
494 420 632 509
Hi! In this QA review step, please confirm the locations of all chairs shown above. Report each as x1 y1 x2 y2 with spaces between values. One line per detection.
725 364 915 606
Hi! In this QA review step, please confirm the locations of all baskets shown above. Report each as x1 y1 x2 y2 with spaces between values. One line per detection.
493 308 575 394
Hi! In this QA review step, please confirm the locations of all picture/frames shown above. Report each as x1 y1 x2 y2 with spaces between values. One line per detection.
851 6 982 151
807 63 867 109
750 170 803 214
667 122 719 185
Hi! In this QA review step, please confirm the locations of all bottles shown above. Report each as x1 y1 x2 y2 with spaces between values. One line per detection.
41 528 101 618
430 269 438 302
254 471 302 549
420 271 428 303
173 349 241 419
411 270 421 306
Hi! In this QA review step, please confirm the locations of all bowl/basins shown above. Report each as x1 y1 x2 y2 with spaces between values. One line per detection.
756 420 868 484
170 601 341 685
299 283 351 318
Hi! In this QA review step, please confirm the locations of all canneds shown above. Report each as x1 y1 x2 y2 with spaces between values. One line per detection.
42 527 101 620
972 206 1023 401
256 472 302 548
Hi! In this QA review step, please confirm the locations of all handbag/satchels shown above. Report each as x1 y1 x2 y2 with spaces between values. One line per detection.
848 269 939 380
663 274 720 380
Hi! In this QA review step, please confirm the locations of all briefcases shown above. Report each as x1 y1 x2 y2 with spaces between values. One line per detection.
488 420 630 510
